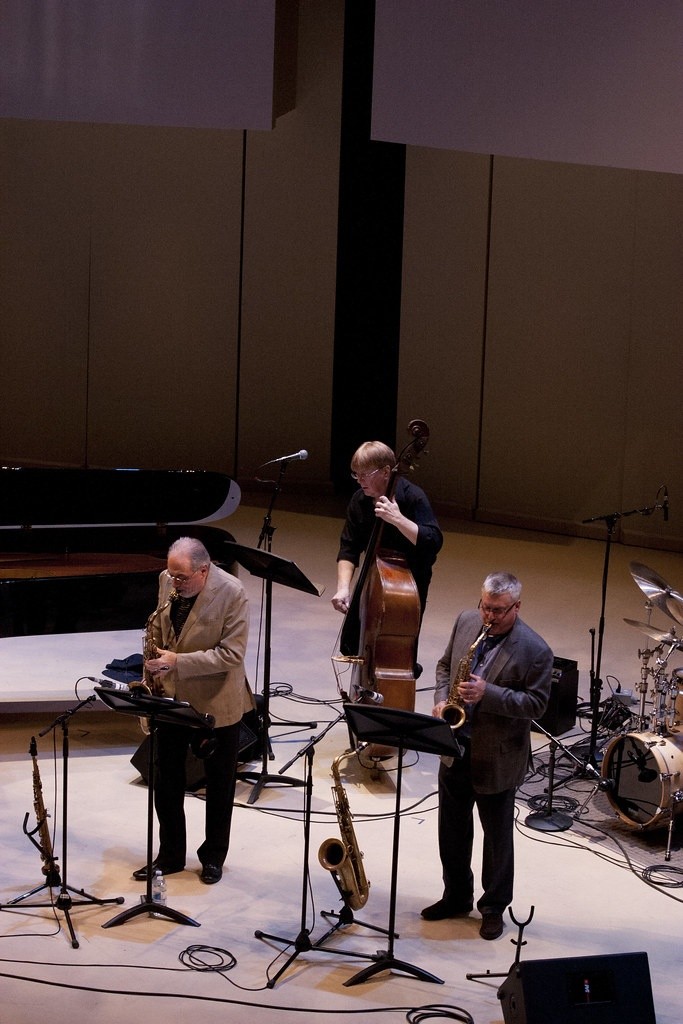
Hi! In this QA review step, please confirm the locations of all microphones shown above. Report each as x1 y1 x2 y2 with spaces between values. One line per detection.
90 678 130 692
269 450 308 463
597 778 616 791
353 684 384 704
663 487 668 522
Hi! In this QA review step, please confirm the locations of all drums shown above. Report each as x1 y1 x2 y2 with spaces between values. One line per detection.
600 730 683 831
666 667 683 735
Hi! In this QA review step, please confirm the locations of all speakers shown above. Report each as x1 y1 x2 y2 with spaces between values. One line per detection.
497 952 656 1024
131 706 265 793
530 656 579 737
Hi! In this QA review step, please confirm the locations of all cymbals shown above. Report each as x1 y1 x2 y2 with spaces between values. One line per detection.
623 617 683 652
627 561 683 626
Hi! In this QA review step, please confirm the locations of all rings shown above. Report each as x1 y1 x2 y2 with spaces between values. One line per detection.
469 694 470 698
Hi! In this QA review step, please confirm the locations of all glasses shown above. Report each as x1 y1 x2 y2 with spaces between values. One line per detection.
351 468 379 480
164 569 201 585
477 598 517 618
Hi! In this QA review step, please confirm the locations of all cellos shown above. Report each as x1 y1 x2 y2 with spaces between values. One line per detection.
329 420 431 781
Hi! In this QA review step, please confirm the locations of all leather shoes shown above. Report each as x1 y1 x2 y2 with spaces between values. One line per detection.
200 863 222 882
421 899 471 920
479 914 503 940
133 859 185 878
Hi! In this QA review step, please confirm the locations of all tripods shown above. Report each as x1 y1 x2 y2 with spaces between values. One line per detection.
254 699 365 989
234 460 317 804
342 702 462 987
0 694 125 950
95 681 216 929
540 502 664 792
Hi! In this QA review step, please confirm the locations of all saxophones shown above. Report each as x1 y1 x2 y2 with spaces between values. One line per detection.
28 735 60 876
318 741 372 911
438 624 494 729
125 590 181 735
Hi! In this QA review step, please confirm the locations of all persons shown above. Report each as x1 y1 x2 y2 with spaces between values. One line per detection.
132 535 254 884
420 571 553 941
330 442 443 763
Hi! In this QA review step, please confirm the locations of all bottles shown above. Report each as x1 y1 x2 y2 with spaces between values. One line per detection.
155 875 168 916
152 871 165 901
